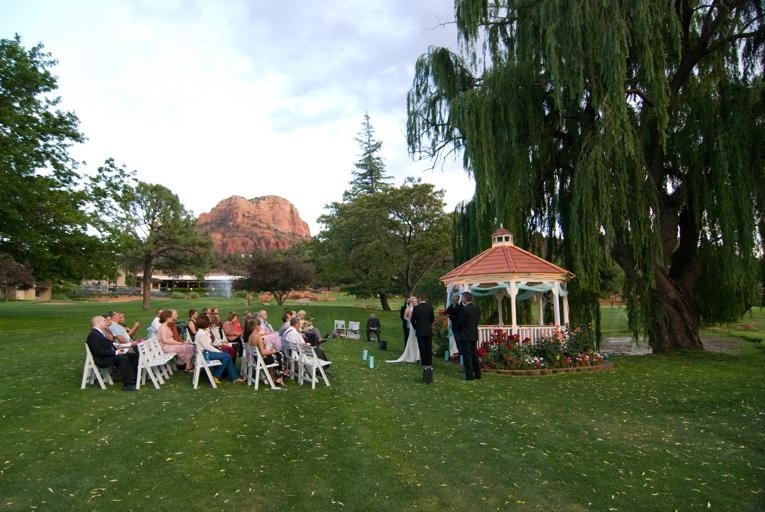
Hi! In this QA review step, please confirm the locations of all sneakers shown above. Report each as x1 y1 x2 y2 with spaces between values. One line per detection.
232 378 244 383
316 371 332 378
275 375 285 386
213 377 221 384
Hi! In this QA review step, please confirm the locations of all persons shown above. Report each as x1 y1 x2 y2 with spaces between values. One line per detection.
385 296 421 363
439 295 463 355
87 307 335 391
411 292 434 370
367 312 381 341
400 298 411 347
452 292 481 380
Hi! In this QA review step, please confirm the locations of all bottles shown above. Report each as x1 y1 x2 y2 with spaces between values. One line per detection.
362 350 368 361
459 355 464 366
369 356 375 369
204 349 209 363
445 350 448 360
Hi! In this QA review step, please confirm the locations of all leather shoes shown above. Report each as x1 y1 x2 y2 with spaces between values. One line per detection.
123 384 136 391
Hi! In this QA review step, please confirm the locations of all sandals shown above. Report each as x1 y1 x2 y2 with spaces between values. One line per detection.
185 367 194 374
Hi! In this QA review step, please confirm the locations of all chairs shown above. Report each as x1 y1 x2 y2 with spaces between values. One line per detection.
80 327 333 391
333 319 379 342
